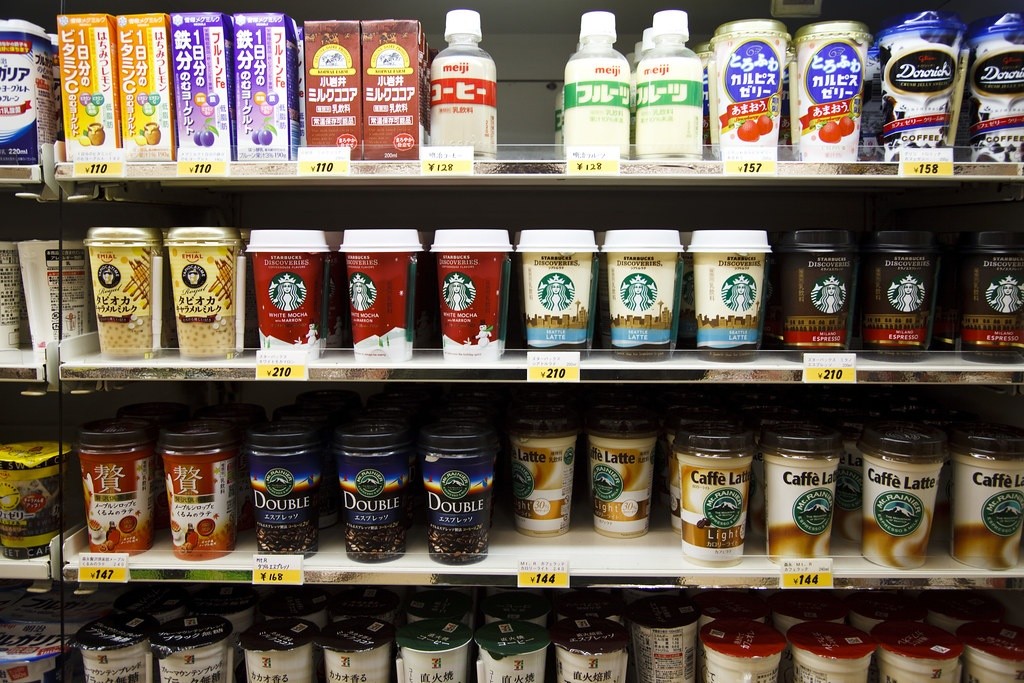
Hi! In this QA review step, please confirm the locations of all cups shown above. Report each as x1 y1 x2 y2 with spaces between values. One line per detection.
72 389 1024 567
0 227 1024 365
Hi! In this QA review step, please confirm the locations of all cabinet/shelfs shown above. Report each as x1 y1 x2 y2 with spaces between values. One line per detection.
0 0 1024 683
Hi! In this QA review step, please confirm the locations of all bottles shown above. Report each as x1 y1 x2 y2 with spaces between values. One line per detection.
555 10 705 160
430 10 498 161
